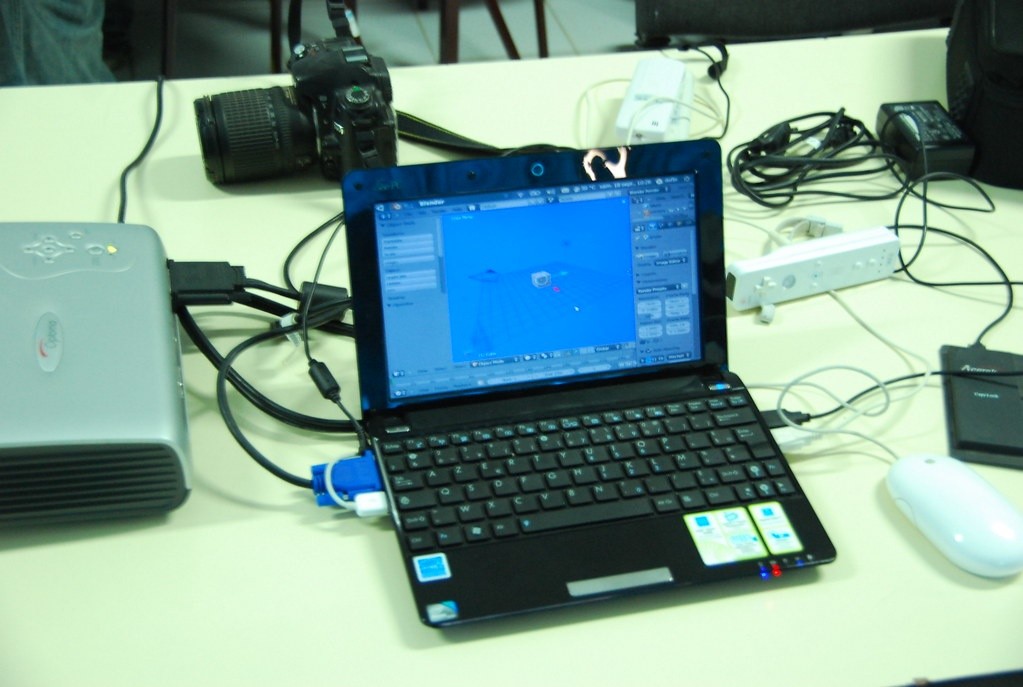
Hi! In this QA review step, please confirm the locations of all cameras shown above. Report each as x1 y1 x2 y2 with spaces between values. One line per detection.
193 35 397 184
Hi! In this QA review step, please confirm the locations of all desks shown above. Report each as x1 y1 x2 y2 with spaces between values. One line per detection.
2 31 1023 687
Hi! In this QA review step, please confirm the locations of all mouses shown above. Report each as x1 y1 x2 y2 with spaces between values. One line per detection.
886 453 1021 577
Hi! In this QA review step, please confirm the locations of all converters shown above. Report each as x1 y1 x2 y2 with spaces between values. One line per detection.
876 101 974 183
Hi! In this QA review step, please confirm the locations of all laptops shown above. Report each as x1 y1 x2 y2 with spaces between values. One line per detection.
341 134 837 627
